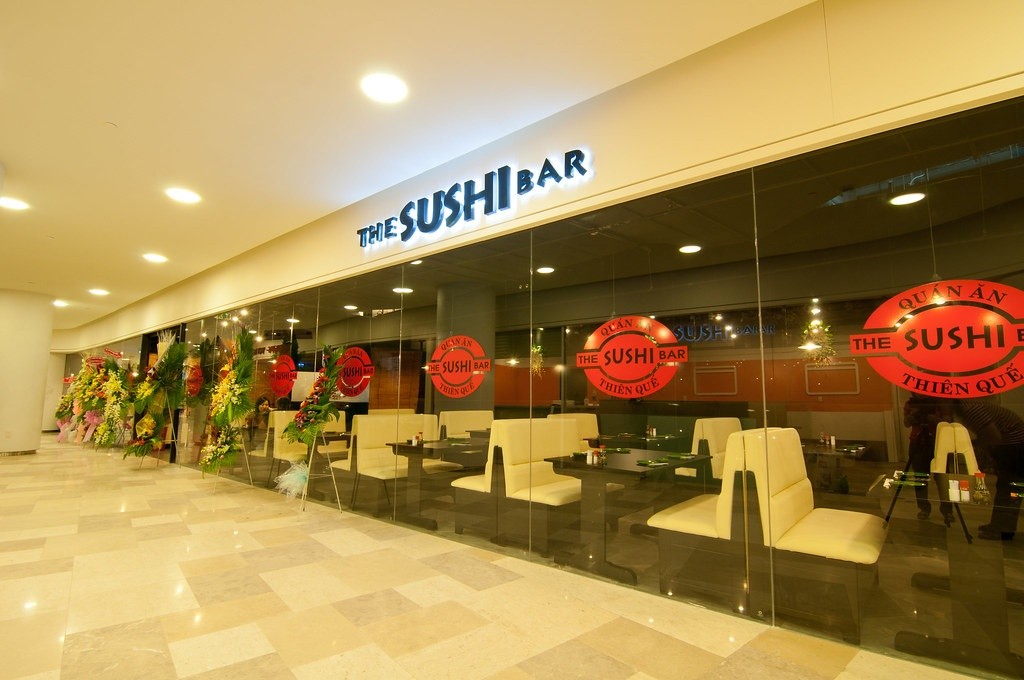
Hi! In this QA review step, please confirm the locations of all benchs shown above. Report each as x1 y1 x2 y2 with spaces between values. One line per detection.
246 407 992 646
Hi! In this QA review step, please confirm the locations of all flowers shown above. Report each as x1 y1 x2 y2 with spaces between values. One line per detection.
281 345 343 447
56 339 188 457
199 329 253 474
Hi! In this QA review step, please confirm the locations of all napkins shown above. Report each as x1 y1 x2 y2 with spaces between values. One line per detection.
884 479 894 488
893 470 902 478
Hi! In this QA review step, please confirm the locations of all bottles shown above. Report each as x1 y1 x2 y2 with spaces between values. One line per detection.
584 396 589 406
960 480 970 501
818 432 835 445
592 390 597 401
586 446 607 465
973 473 990 504
949 480 960 501
412 432 423 447
646 425 656 437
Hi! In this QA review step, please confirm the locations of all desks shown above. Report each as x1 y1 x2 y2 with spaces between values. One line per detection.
881 469 1024 679
801 442 870 492
465 427 490 439
544 448 714 586
300 431 350 499
386 438 489 531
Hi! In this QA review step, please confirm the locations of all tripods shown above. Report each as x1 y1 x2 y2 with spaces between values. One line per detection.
882 411 973 545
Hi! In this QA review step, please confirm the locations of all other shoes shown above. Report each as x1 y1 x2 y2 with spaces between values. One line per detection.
918 509 931 519
978 524 994 529
942 512 955 523
978 529 1015 541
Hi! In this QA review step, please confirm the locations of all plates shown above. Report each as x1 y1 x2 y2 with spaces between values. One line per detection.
636 445 692 466
1011 481 1023 487
1013 493 1024 498
572 448 631 457
889 471 930 487
835 444 863 451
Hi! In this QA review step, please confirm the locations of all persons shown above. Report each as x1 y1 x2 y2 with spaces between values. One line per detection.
903 389 956 523
256 397 291 450
246 398 274 428
953 399 1024 540
